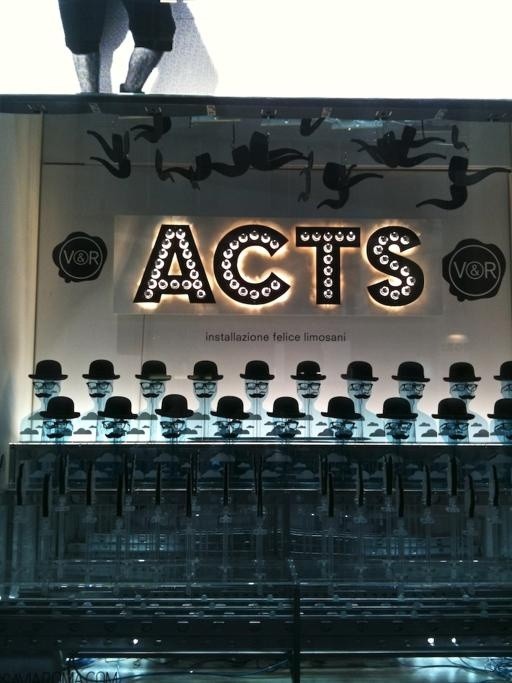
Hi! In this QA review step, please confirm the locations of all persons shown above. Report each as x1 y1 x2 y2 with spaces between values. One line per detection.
56 0 176 95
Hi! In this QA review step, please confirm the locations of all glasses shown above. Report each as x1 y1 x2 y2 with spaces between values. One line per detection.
275 420 298 429
329 422 510 431
102 421 131 428
298 382 320 389
43 420 73 428
160 419 185 431
214 421 242 430
32 382 60 389
246 382 269 390
140 382 164 389
193 382 216 389
87 382 111 390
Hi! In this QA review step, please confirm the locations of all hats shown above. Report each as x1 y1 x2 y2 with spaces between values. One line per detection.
267 396 306 418
135 360 172 380
28 358 67 380
81 359 122 379
98 397 139 420
39 396 80 420
290 359 326 381
239 359 275 380
210 395 249 419
154 394 195 418
187 360 224 380
322 360 511 420
